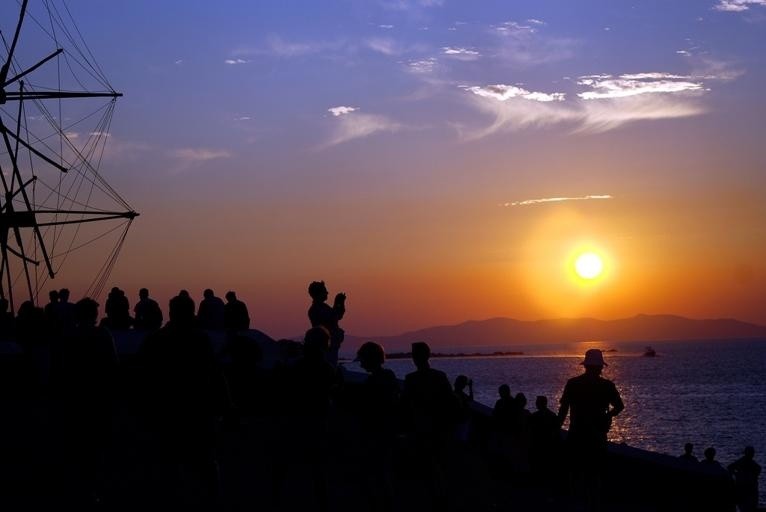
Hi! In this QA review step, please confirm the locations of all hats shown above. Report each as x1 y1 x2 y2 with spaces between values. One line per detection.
109 287 124 297
579 349 608 366
352 342 384 362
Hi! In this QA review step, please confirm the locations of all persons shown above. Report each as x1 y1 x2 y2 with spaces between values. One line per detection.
680 442 761 480
495 347 624 446
354 341 476 399
302 278 345 361
0 288 250 337
